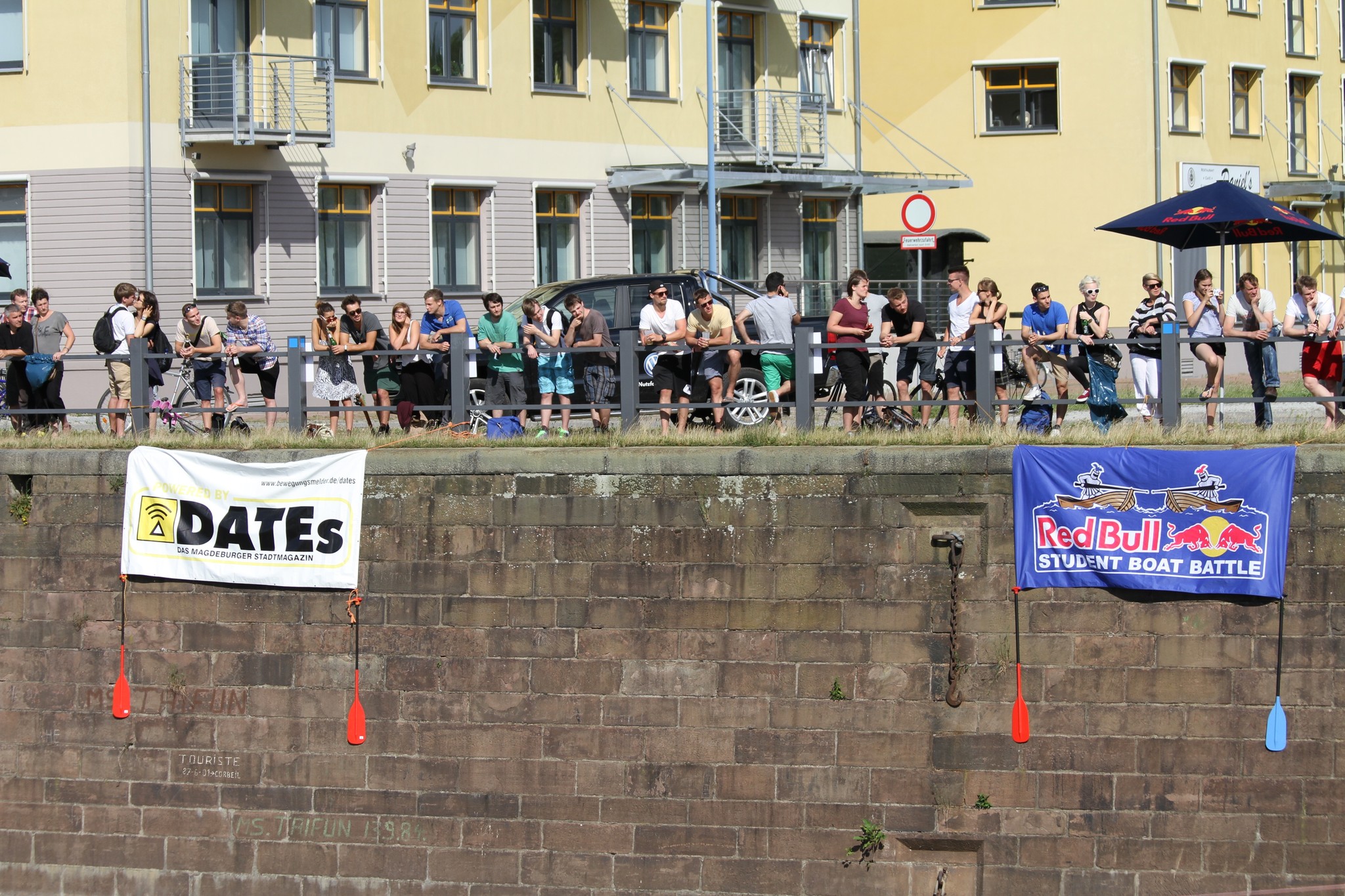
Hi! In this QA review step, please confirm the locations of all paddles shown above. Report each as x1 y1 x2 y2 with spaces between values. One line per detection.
1264 591 1289 753
346 595 364 745
1010 585 1029 742
111 574 130 717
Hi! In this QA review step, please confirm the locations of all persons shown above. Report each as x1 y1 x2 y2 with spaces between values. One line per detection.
0 267 1345 436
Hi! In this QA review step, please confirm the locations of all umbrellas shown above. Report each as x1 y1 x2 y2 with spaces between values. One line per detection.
1095 180 1345 432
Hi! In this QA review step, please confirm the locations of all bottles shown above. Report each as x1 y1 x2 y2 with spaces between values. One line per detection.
1308 313 1320 339
230 343 240 367
162 349 169 365
1081 310 1089 335
327 332 337 355
184 335 191 359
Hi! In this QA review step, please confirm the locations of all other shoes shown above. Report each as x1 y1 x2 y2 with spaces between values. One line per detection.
1265 387 1278 397
769 389 781 417
1076 388 1090 402
1023 388 1041 401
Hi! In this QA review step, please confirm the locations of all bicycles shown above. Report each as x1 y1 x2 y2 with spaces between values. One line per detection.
94 358 234 437
822 351 897 429
908 348 1047 416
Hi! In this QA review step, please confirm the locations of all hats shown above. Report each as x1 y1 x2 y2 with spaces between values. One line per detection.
647 280 666 299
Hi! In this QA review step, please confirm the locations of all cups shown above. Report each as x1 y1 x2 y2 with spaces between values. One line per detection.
751 340 761 355
1259 322 1268 340
702 331 710 347
644 330 653 345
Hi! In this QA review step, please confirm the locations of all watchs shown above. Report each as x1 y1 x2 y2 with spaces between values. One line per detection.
661 334 666 341
140 317 146 321
1088 318 1094 324
491 342 495 345
957 336 963 341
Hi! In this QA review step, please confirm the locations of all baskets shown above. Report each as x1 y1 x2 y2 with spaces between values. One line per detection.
1007 350 1043 377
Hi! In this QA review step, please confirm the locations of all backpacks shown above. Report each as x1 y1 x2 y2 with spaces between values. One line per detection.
881 406 920 431
526 306 570 335
92 304 127 354
1017 392 1053 439
151 318 173 374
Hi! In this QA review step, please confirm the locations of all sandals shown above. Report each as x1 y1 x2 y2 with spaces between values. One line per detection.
1200 383 1214 399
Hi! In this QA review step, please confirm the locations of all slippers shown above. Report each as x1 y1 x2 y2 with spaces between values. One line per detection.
722 397 734 404
225 401 248 411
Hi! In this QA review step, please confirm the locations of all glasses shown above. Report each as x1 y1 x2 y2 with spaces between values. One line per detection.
697 299 713 308
347 308 362 315
321 314 337 322
652 290 668 296
948 279 961 282
136 295 145 304
395 310 405 315
1036 285 1049 292
183 303 197 316
977 289 986 294
1145 282 1162 289
1083 288 1100 294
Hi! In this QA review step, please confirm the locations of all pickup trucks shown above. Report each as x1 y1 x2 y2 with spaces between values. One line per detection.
436 271 846 431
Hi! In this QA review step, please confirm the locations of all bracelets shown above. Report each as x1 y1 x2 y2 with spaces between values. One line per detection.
63 347 69 353
345 344 347 352
1218 301 1223 304
1077 334 1082 344
668 341 671 345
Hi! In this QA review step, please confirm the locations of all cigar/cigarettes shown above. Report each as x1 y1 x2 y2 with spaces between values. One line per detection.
229 353 231 355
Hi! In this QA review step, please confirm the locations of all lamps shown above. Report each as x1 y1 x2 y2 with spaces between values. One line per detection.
1329 163 1338 173
402 143 416 157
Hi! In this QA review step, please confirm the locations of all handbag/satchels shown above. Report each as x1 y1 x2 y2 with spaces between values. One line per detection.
230 416 250 437
486 416 523 442
304 422 336 444
1101 329 1122 369
